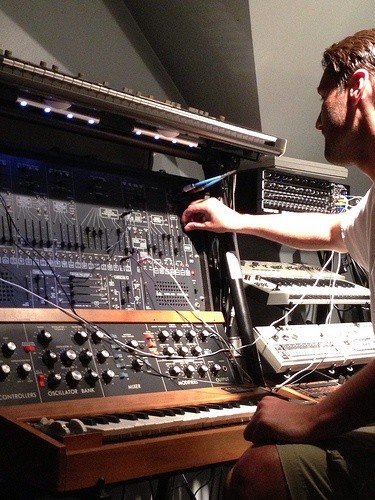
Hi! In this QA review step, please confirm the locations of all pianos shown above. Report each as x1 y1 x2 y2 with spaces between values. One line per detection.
0 307 319 495
243 274 370 304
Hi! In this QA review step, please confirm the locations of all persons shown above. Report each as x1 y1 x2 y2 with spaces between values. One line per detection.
180 28 375 500
